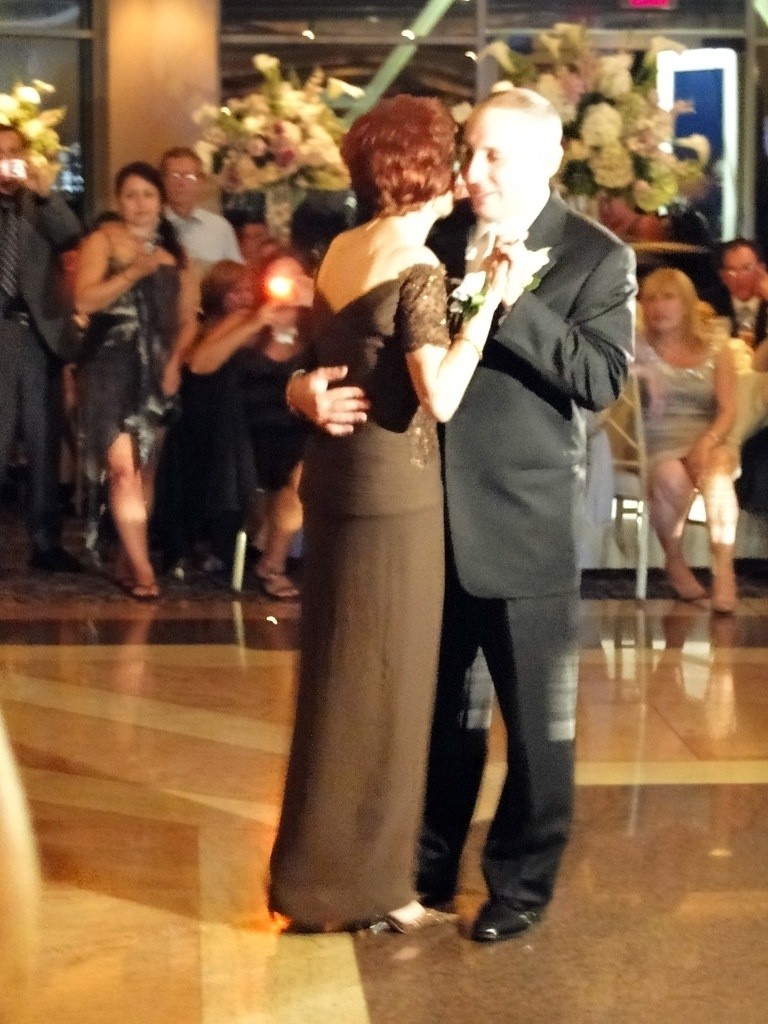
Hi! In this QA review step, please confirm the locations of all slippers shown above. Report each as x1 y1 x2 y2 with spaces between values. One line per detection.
113 574 137 595
131 577 160 601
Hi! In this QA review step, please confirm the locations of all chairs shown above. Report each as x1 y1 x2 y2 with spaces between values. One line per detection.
601 373 742 604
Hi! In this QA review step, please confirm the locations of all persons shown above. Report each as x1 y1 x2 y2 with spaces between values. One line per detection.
268 88 637 943
0 125 768 616
268 93 530 931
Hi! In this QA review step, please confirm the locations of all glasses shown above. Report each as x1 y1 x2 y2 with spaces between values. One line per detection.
725 264 754 276
165 171 201 184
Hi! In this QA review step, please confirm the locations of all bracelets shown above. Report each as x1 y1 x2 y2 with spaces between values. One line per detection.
452 333 483 362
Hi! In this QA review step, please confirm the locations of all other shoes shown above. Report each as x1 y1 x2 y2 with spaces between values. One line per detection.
664 559 706 600
31 544 83 580
711 573 737 614
366 899 462 934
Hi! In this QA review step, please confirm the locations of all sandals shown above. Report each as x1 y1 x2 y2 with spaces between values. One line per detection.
256 566 300 600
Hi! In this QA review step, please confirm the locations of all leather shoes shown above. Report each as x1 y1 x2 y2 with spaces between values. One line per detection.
472 897 544 941
413 890 456 909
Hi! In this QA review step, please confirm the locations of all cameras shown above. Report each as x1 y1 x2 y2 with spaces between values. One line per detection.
0 159 28 179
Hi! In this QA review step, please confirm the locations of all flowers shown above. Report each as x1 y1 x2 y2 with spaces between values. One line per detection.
190 53 365 245
450 18 711 225
0 78 80 192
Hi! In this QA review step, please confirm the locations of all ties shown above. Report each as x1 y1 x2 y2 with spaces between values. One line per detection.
0 199 20 298
458 229 497 300
739 306 754 334
